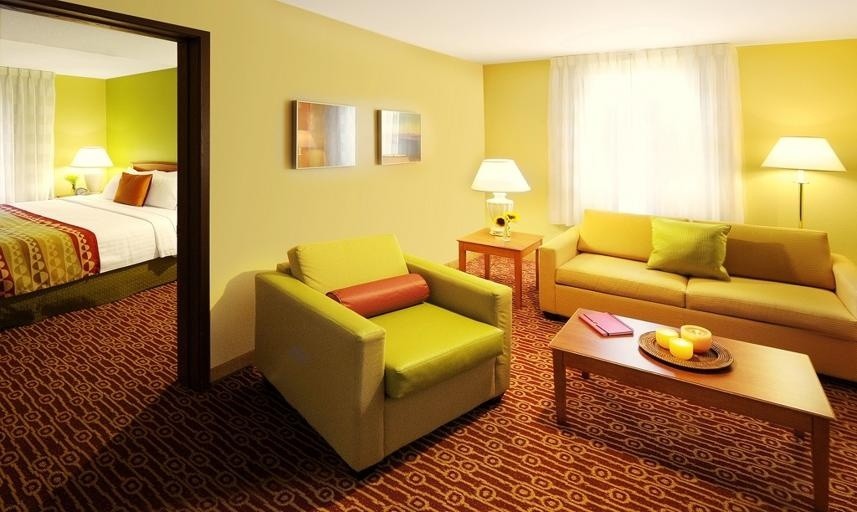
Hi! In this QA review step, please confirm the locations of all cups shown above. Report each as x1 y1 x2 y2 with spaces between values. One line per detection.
655 325 715 358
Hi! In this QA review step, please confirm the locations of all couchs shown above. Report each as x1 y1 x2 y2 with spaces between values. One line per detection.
536 201 857 381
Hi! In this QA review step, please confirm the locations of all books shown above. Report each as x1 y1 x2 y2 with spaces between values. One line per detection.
578 310 634 337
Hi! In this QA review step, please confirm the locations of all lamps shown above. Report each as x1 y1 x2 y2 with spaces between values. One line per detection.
469 158 532 238
64 143 114 194
753 130 849 227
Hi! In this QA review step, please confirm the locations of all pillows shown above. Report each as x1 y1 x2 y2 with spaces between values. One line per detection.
576 208 689 263
693 220 835 291
646 212 732 283
106 168 178 210
287 230 409 296
326 273 431 318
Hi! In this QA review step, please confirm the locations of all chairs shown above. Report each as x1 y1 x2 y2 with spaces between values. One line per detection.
253 231 513 478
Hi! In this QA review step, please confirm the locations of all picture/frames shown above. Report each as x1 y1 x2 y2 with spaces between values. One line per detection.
290 99 358 171
376 109 423 169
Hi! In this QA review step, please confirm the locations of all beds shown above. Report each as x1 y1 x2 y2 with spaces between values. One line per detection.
0 160 179 329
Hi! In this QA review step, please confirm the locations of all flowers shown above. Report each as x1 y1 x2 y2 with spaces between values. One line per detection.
493 210 520 242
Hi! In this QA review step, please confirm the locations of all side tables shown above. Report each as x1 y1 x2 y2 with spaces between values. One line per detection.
457 223 543 310
